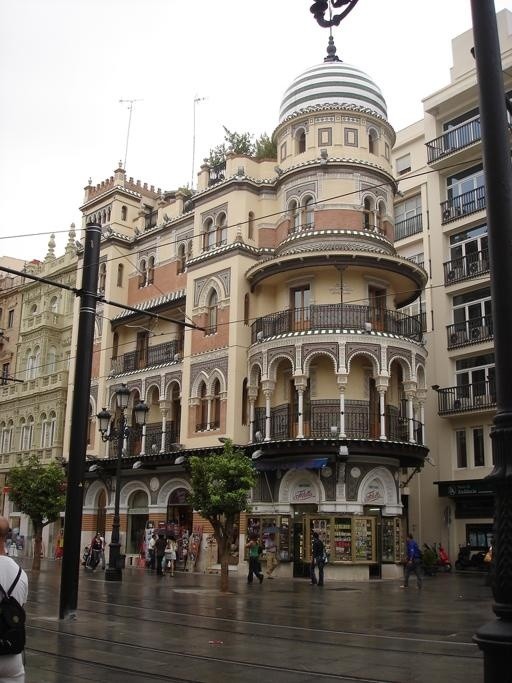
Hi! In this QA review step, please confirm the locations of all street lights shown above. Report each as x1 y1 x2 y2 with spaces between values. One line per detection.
97 381 149 582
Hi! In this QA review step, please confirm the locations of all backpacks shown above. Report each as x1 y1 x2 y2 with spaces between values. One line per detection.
0 579 26 655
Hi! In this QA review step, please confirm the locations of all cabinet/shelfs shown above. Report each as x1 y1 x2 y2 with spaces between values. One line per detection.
302 514 377 566
381 517 402 564
245 515 294 563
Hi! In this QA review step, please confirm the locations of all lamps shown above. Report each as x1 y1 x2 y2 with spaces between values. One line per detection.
273 165 284 176
237 165 244 177
252 446 264 460
256 331 264 342
174 455 188 465
321 149 328 164
109 370 115 378
132 461 144 470
173 353 181 363
331 427 337 438
162 213 171 223
184 200 194 213
76 240 83 249
170 442 184 451
255 431 264 443
364 322 372 332
339 443 349 456
218 438 230 444
134 226 142 235
89 464 112 491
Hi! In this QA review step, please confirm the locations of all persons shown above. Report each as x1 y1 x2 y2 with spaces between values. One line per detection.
265 533 279 580
149 535 177 577
57 529 64 560
245 533 263 585
0 517 28 683
399 533 424 591
91 529 105 572
309 532 326 587
4 528 24 556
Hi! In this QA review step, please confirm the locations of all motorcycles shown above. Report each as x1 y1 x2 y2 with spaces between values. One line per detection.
422 541 452 573
455 543 490 571
82 540 106 569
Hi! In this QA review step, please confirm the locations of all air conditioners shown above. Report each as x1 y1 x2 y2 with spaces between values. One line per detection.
474 395 491 406
443 207 458 221
469 260 487 275
471 327 489 340
452 397 469 410
447 267 463 282
449 331 467 346
